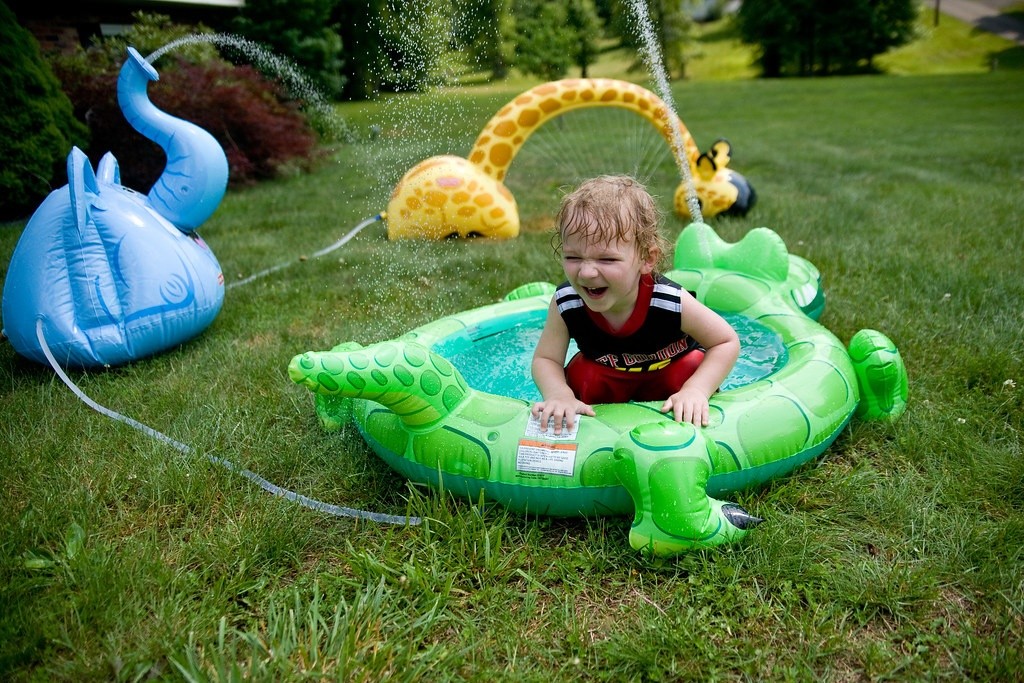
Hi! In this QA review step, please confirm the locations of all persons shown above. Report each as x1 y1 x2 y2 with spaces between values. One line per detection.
531 173 741 435
